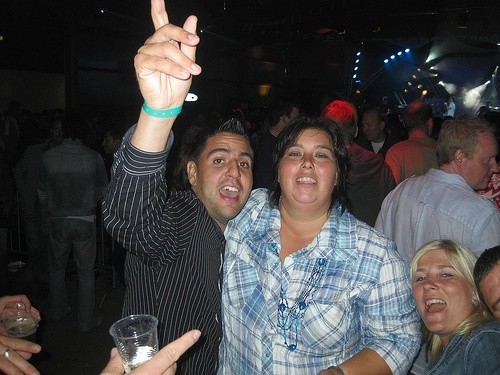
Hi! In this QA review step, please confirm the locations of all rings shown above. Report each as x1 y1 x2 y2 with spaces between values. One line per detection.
3 348 12 359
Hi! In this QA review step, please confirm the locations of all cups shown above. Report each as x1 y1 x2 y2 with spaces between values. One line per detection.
2 301 39 343
109 314 159 374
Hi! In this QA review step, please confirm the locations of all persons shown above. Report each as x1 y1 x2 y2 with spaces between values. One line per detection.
100 0 252 375
0 0 500 375
218 117 420 375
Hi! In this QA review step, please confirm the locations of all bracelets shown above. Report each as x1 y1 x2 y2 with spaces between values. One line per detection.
328 365 345 375
143 92 198 118
